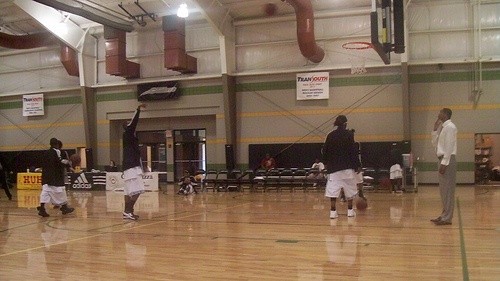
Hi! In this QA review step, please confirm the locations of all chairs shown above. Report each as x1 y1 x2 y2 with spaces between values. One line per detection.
194 169 394 190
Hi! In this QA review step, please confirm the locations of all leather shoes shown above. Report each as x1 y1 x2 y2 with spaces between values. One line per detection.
430 217 452 225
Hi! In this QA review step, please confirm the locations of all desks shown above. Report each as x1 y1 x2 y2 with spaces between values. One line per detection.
16 173 167 192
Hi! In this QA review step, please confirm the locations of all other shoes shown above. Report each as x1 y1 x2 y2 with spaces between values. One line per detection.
391 189 404 193
313 183 318 187
8 195 12 200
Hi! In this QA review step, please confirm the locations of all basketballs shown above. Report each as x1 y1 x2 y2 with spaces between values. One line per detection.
357 197 369 211
71 154 82 166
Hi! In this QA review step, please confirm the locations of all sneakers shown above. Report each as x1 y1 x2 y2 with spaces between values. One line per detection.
347 208 356 217
123 208 139 220
330 210 339 219
38 209 49 217
62 207 74 215
340 196 346 202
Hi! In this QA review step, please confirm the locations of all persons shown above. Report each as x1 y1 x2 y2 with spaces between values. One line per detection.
0 155 12 200
430 106 458 225
121 102 146 221
37 138 74 217
177 169 198 195
110 160 117 170
312 116 368 219
261 151 276 171
387 143 403 194
